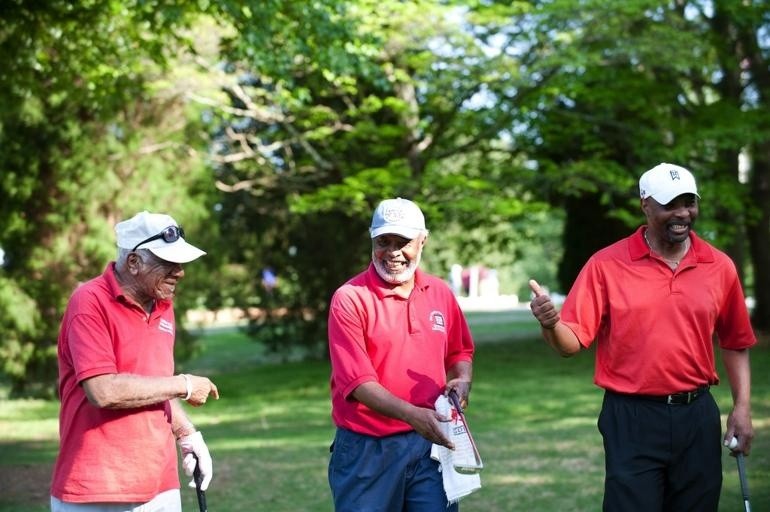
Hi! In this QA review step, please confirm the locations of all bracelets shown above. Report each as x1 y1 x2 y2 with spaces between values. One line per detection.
174 423 194 437
179 373 192 401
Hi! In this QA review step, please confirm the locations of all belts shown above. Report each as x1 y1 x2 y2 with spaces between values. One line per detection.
640 387 711 405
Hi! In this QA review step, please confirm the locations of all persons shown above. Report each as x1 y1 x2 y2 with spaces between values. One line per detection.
326 199 476 511
49 211 220 512
527 164 757 511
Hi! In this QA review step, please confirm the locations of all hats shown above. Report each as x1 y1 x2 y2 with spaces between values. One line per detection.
115 212 207 265
371 197 425 241
639 163 702 206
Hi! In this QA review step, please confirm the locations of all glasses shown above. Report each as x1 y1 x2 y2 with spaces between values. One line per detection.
131 226 185 251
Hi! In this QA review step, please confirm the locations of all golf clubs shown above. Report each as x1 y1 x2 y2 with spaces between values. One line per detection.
449 391 484 475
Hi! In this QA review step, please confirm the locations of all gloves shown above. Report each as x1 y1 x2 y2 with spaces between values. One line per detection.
178 431 212 490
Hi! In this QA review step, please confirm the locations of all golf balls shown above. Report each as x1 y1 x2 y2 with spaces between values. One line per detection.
728 436 737 450
